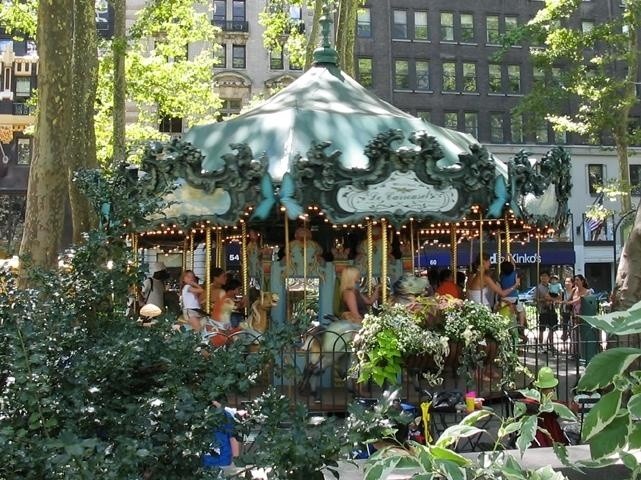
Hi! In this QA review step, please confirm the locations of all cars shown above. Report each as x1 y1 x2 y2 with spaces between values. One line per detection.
518 286 536 302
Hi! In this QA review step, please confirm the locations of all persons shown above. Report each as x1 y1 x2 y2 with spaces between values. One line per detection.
339 267 383 319
534 270 591 354
435 251 528 339
140 261 166 309
181 268 247 324
